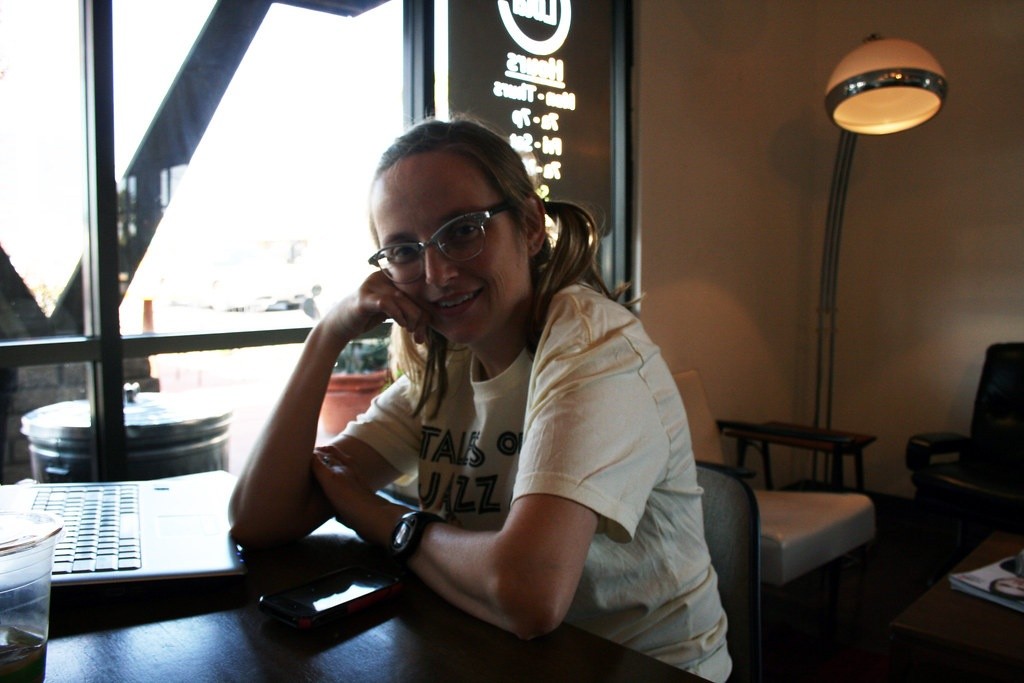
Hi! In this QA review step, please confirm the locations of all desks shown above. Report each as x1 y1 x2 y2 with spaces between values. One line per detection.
891 532 1024 681
736 422 877 495
0 517 714 683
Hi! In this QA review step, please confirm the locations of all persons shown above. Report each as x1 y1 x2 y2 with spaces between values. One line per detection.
227 118 733 683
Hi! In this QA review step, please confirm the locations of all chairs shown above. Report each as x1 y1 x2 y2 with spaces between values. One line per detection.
905 341 1024 593
669 370 876 683
696 460 762 683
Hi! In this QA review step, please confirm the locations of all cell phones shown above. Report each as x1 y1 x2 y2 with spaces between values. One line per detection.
260 560 410 628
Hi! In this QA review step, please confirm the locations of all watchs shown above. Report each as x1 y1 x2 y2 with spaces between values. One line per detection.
388 511 448 564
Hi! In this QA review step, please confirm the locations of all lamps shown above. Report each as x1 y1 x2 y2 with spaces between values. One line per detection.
810 30 948 485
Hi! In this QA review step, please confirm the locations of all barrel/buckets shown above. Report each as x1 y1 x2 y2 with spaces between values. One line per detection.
19 382 235 485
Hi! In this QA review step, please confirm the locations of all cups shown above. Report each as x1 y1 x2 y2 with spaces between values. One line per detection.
0 510 63 673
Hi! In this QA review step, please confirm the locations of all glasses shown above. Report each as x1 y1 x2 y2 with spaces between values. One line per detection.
369 202 511 283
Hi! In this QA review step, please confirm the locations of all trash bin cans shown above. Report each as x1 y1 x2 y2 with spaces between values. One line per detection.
19 382 229 484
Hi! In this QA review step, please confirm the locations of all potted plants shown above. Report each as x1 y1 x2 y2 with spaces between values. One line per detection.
322 341 392 433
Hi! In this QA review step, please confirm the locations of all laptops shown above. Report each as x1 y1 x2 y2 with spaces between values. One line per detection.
0 445 249 595
